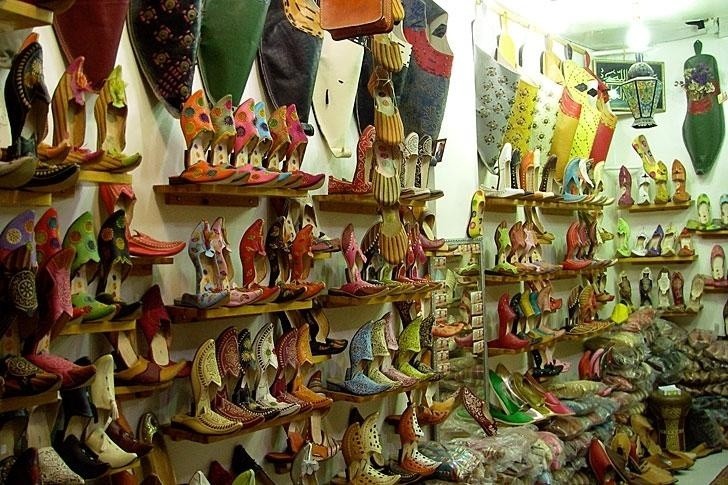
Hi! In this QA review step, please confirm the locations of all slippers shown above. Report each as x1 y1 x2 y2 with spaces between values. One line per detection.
682 55 724 176
585 414 696 484
0 43 463 485
473 20 618 182
481 136 728 346
581 350 612 380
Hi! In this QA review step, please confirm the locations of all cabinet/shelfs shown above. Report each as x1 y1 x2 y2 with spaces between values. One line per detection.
617 195 727 321
0 1 440 485
426 246 483 379
480 181 613 358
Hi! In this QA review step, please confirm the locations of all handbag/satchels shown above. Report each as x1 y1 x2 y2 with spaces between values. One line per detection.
319 0 393 40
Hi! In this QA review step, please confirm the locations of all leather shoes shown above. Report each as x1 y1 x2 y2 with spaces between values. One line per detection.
467 190 485 239
465 364 577 436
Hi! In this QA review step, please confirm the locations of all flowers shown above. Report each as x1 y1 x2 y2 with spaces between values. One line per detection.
679 71 717 98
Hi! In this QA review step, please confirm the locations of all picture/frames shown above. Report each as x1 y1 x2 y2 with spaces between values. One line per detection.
592 57 668 115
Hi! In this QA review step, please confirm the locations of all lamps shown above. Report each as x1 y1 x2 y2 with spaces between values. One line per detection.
619 51 660 131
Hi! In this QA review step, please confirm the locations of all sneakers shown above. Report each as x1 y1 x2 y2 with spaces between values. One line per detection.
355 23 414 131
200 1 269 107
401 0 453 141
55 0 129 89
128 0 202 114
261 0 324 133
391 0 405 21
312 24 365 155
